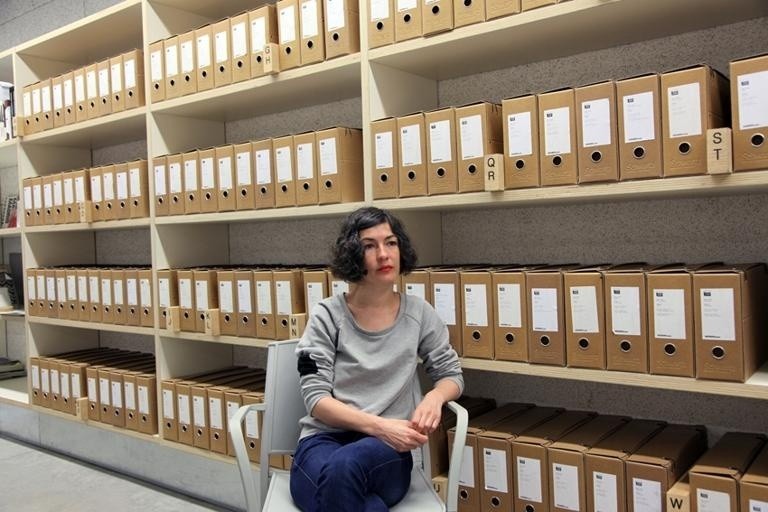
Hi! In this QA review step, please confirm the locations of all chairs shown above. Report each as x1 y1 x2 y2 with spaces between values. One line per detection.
228 334 469 512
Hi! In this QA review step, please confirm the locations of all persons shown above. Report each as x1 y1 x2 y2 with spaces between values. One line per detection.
287 204 467 512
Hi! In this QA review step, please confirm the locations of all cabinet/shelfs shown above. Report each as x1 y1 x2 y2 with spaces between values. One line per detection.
0 1 768 512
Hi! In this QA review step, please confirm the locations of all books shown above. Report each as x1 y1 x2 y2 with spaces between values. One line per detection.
0 357 27 380
